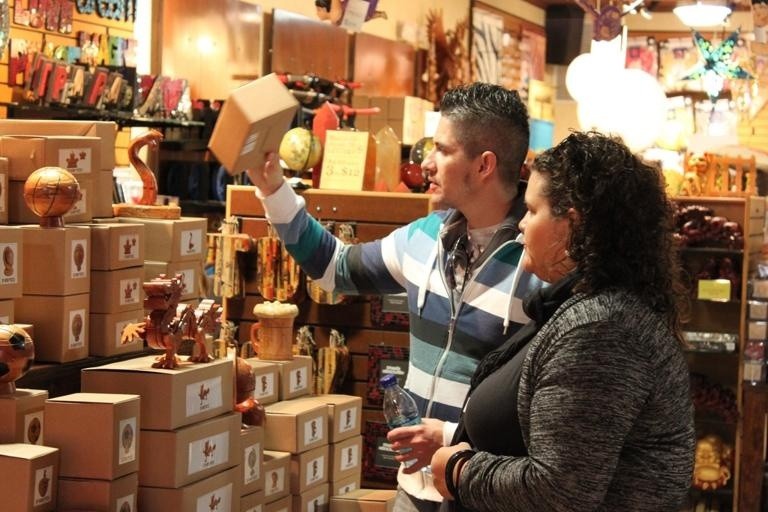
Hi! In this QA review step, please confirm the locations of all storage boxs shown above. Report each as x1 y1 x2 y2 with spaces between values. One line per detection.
261 402 329 452
42 392 141 481
137 466 241 512
9 174 89 224
87 268 145 313
0 226 23 301
0 443 60 512
250 354 315 400
207 72 299 175
91 313 146 355
331 434 365 478
238 424 264 495
14 224 90 296
349 95 434 142
295 393 361 445
261 448 288 504
117 215 209 263
330 472 363 497
137 259 204 300
0 389 48 444
262 495 292 511
0 134 101 182
293 482 330 512
2 119 117 171
0 296 13 328
10 291 91 364
56 473 139 512
137 411 241 487
331 488 397 512
289 445 330 495
88 171 113 220
239 489 265 511
81 353 234 433
90 222 143 271
242 359 281 403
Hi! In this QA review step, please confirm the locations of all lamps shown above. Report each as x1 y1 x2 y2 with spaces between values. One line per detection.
673 0 734 26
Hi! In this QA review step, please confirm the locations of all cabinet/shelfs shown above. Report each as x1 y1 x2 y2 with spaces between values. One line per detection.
666 194 753 509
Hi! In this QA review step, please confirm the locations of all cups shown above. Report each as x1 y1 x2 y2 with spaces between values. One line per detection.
250 317 295 360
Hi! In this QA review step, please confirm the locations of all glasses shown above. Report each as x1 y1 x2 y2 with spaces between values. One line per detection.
442 231 470 295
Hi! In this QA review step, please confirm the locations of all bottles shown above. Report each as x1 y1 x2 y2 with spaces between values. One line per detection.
379 374 431 474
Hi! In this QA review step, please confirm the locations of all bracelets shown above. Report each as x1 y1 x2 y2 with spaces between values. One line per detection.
445 450 478 502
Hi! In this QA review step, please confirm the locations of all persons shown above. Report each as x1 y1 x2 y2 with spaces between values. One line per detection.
245 81 554 512
429 128 697 512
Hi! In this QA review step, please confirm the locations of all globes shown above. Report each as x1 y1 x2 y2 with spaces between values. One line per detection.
280 125 322 189
